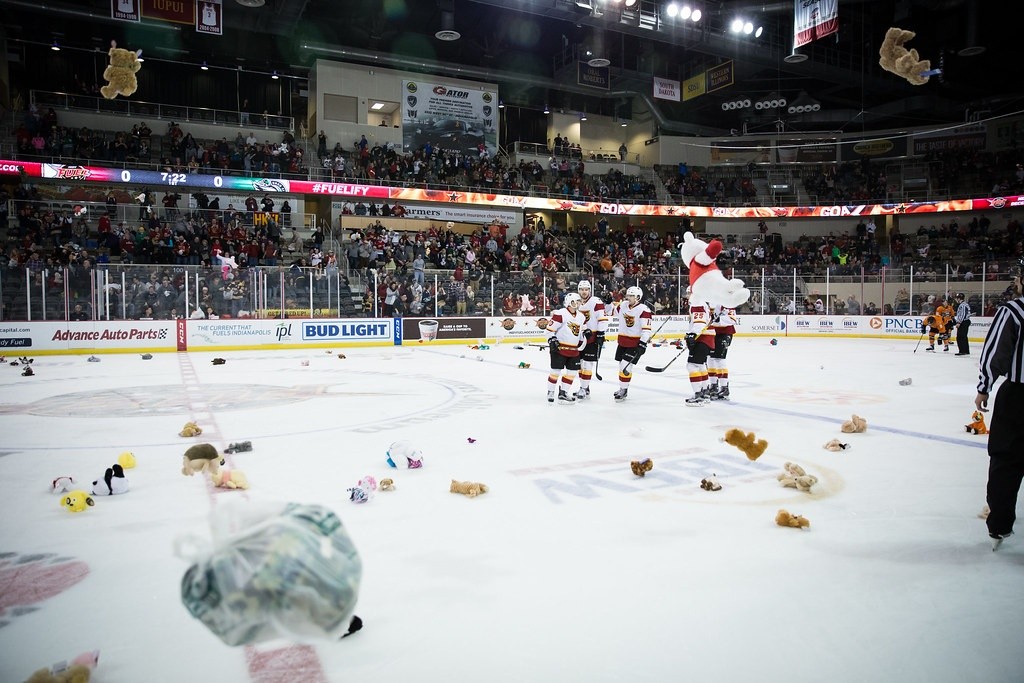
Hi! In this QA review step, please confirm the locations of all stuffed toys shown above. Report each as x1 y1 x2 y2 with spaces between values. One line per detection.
178 421 254 488
965 411 990 434
0 355 35 376
88 350 346 369
679 232 750 308
344 441 487 502
101 47 141 98
472 344 530 369
770 339 777 345
880 28 929 84
53 451 135 512
631 416 866 528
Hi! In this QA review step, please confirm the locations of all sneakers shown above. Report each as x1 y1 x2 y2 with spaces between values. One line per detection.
613 387 627 402
987 531 1013 552
572 386 590 402
709 379 730 401
547 391 555 402
926 343 935 352
558 384 576 405
942 344 949 351
684 384 711 408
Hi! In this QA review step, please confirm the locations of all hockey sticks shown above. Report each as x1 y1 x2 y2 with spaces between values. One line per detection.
595 340 604 381
622 314 672 376
528 335 588 352
645 308 726 373
913 334 923 353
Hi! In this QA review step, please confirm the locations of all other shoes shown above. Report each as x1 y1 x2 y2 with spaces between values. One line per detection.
955 351 970 355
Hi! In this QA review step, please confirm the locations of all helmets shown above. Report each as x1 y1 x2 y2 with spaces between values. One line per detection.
577 280 592 292
954 292 966 300
626 286 643 299
562 292 582 307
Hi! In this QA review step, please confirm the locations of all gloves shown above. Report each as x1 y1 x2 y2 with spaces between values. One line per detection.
686 332 697 340
595 330 605 342
601 291 613 305
584 329 592 339
636 340 647 355
547 336 559 353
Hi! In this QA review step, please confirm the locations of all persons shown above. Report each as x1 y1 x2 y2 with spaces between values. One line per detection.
545 293 585 402
0 83 1024 355
601 286 652 402
709 306 736 398
574 281 608 399
976 257 1024 551
685 285 714 406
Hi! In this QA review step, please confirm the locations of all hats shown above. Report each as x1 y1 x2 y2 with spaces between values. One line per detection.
927 316 936 324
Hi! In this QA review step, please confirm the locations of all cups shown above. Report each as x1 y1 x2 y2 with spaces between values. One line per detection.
418 320 438 341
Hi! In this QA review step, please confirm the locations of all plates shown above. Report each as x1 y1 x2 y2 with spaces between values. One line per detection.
537 318 548 329
503 318 514 330
